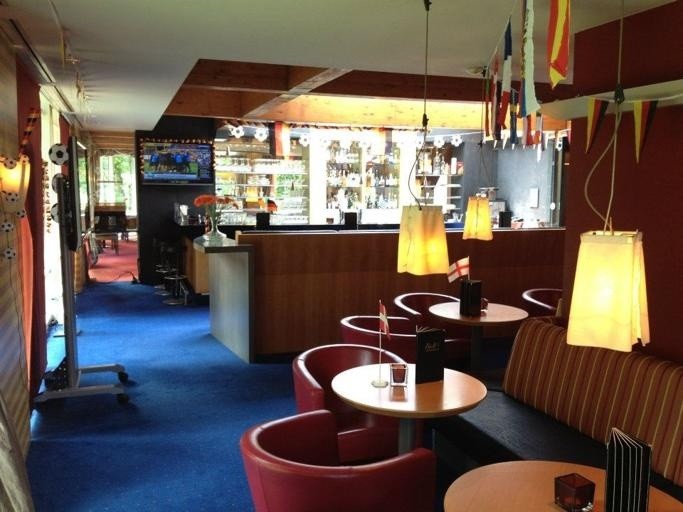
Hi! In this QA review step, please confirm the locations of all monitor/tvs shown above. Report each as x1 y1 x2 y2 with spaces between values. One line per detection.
68 135 82 252
138 136 215 188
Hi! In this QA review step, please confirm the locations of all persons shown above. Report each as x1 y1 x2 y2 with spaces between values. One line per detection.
149 148 191 174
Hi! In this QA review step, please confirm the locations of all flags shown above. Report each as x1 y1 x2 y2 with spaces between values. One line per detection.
380 303 392 341
586 98 610 156
447 257 469 283
633 100 659 164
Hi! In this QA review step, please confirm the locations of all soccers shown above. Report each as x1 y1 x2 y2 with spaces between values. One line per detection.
3 247 16 259
4 157 17 169
15 208 26 218
6 191 19 202
51 203 59 223
230 126 244 137
52 173 66 193
450 134 463 147
298 134 310 147
1 221 14 233
48 142 70 166
20 154 29 164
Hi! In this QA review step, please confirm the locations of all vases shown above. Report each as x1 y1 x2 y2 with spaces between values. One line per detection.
203 212 227 241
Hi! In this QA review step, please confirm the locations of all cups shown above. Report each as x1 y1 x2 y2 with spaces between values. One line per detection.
281 215 308 225
217 157 253 171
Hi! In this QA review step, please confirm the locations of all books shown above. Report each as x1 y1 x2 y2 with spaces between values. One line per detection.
415 325 446 384
606 426 653 512
459 279 482 317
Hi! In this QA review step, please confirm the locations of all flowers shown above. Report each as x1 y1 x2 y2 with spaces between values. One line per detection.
451 134 463 147
231 126 245 139
297 134 312 147
194 193 239 237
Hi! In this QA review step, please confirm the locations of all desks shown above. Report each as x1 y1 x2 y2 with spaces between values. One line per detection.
193 227 566 364
331 363 488 449
428 302 529 377
444 460 683 512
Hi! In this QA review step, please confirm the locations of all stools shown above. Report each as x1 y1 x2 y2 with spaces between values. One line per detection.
153 233 183 296
161 241 188 305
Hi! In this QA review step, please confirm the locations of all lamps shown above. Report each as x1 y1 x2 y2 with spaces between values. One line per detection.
566 230 651 353
397 206 451 276
462 195 493 241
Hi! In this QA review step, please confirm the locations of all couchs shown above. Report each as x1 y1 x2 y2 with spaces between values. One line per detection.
340 316 464 372
239 409 436 512
292 343 408 462
521 288 562 317
392 292 460 335
423 315 683 502
